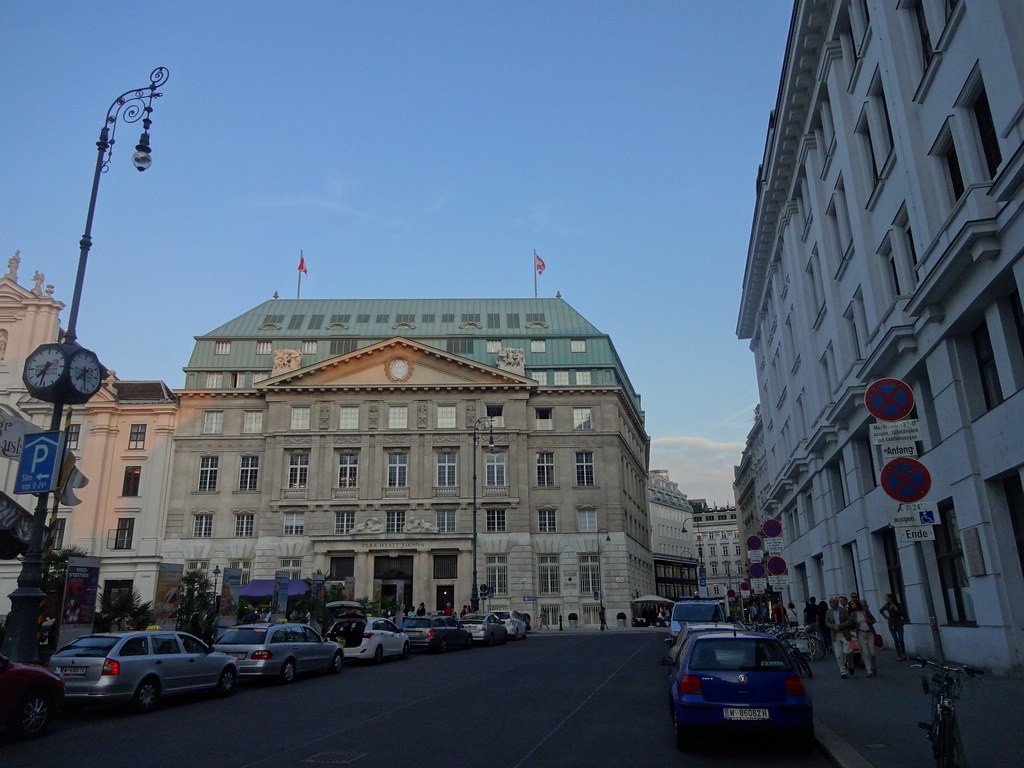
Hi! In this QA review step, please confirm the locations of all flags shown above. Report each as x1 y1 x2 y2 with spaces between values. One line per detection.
298 252 307 275
535 253 546 276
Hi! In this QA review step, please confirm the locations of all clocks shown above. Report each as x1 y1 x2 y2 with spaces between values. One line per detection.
157 640 173 654
22 343 67 387
67 348 101 394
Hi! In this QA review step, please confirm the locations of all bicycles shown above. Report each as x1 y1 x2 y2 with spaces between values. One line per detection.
751 622 826 677
909 656 984 768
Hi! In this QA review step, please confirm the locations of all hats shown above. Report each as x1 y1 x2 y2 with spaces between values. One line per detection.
419 603 424 606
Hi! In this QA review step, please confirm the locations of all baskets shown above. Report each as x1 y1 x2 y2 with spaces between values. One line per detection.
919 666 967 698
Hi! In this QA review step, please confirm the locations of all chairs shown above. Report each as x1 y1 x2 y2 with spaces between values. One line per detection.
692 646 723 668
742 646 766 668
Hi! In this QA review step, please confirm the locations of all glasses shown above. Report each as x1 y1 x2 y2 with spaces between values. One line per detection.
851 596 857 598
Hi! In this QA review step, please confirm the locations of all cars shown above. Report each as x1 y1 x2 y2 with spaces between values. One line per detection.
459 610 527 647
0 653 64 739
399 616 473 654
212 619 344 685
49 626 240 716
659 620 815 750
325 601 411 665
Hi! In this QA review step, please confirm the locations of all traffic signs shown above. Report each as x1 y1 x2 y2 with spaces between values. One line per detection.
13 431 68 494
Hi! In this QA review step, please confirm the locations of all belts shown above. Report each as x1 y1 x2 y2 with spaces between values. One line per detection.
858 630 871 632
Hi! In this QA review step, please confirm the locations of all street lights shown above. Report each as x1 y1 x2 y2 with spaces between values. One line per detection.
0 65 172 665
681 517 703 578
597 528 611 609
212 565 221 613
680 551 693 596
470 416 495 612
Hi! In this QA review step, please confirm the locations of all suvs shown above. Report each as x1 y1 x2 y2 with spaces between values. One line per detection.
670 599 726 643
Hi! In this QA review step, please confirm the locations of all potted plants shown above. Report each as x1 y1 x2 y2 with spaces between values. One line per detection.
568 613 578 628
617 612 626 628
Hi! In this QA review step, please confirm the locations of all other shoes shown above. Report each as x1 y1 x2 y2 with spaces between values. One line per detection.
902 654 907 659
867 670 872 676
841 672 847 677
847 662 855 675
898 657 901 661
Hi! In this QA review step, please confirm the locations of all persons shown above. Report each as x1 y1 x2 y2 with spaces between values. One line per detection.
880 594 908 663
824 597 855 679
289 608 311 628
536 610 549 630
407 602 472 617
599 608 610 630
849 600 876 678
772 592 863 654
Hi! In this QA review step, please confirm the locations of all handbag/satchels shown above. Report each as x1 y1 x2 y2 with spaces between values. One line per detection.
874 634 883 648
